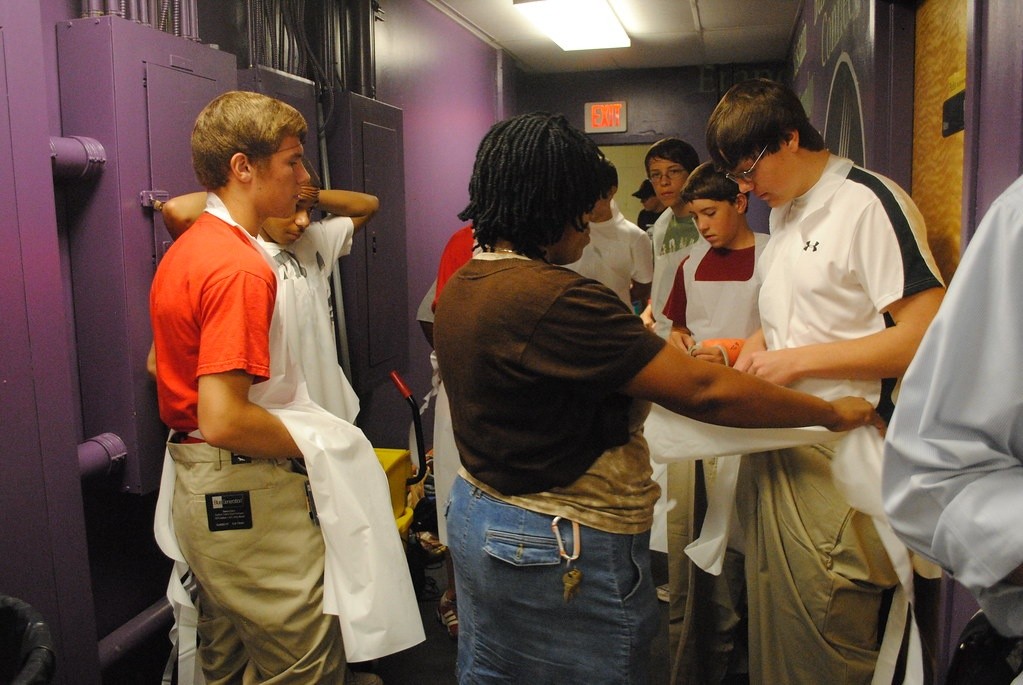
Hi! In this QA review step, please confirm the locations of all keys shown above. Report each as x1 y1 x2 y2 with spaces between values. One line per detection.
563 568 581 602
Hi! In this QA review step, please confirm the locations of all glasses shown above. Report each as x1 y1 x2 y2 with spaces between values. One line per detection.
647 168 687 182
726 145 768 184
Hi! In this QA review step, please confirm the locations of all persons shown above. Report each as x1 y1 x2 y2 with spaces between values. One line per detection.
883 176 1023 685
707 77 947 685
147 91 427 685
417 113 878 685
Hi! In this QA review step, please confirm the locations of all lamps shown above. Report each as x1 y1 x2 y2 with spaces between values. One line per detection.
513 0 631 52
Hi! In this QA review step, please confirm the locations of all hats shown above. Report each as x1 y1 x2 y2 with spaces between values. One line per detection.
631 180 655 199
